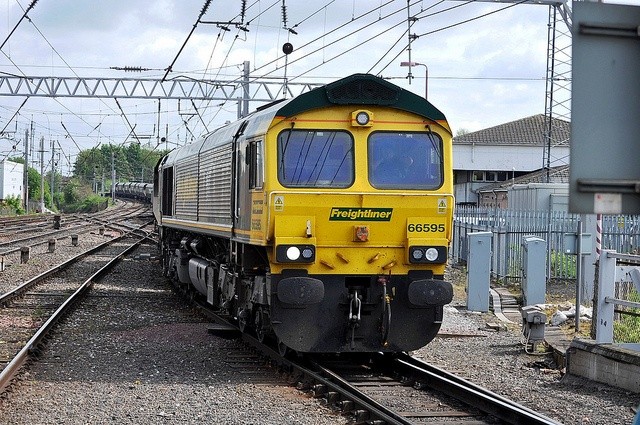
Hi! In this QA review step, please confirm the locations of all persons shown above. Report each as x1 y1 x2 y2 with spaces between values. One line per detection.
374 137 429 183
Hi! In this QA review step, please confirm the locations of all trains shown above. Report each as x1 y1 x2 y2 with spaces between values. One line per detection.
109 73 456 363
